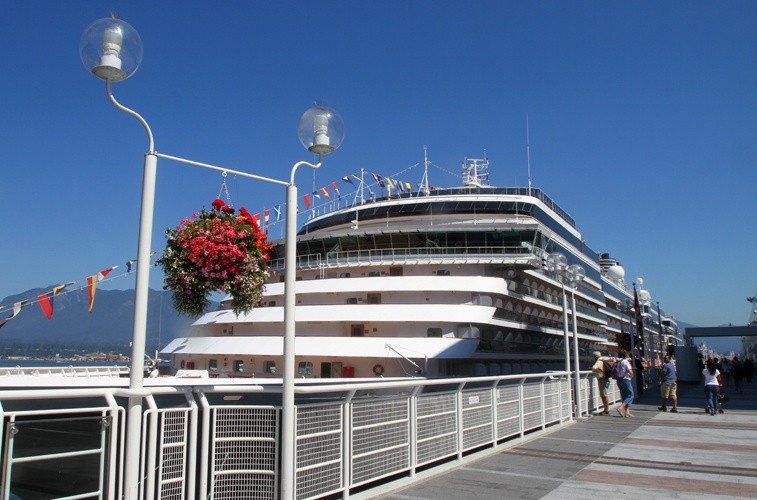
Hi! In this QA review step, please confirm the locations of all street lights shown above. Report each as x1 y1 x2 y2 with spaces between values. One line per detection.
546 252 586 425
615 296 638 399
79 9 345 496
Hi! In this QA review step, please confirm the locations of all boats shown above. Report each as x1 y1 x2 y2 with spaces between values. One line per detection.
0 114 685 405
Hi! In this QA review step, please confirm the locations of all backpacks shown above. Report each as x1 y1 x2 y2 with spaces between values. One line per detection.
597 359 611 378
612 358 626 380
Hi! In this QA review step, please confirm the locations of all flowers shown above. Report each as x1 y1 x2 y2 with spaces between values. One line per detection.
152 199 273 320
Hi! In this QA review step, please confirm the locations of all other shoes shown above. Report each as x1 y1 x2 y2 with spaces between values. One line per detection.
625 413 634 418
599 411 610 416
658 406 667 411
616 407 625 417
670 408 677 413
711 411 714 416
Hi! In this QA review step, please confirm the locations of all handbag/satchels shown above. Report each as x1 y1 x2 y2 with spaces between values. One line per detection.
717 392 726 403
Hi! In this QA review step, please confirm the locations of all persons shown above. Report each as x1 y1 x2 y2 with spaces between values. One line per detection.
698 355 755 392
616 351 635 417
702 359 721 415
591 351 611 415
658 353 678 413
628 353 648 396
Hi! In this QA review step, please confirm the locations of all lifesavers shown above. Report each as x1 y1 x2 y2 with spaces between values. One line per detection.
181 360 185 369
372 364 384 376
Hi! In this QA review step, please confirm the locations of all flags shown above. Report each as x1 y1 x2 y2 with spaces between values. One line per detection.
633 288 644 342
